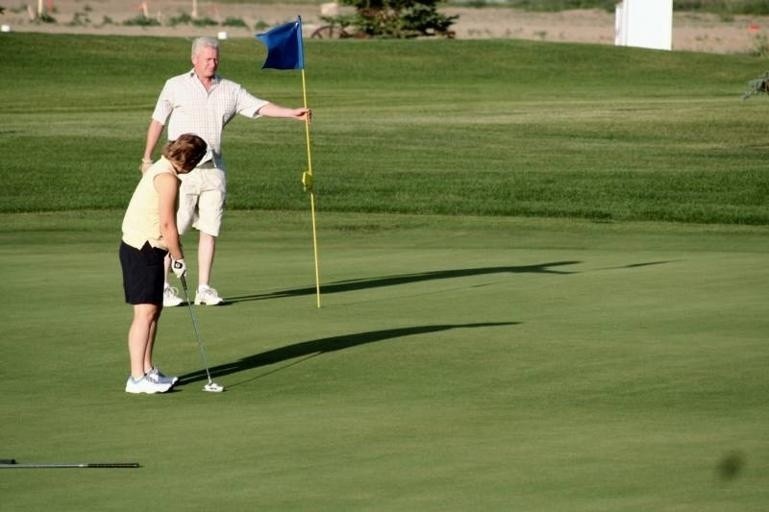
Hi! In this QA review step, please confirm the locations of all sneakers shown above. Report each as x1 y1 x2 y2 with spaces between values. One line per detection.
125 375 173 394
144 368 179 386
194 289 225 306
160 289 184 307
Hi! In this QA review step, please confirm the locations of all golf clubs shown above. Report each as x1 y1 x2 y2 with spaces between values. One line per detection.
176 262 223 393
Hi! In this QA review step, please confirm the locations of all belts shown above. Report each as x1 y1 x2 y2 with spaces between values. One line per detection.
196 161 217 170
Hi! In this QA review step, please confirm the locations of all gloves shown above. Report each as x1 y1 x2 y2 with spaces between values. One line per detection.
141 158 153 174
171 258 188 279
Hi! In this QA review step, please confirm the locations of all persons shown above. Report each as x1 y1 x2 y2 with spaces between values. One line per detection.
138 35 313 308
118 133 208 397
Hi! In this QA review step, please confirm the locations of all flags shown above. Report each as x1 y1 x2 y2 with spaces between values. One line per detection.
253 19 304 70
